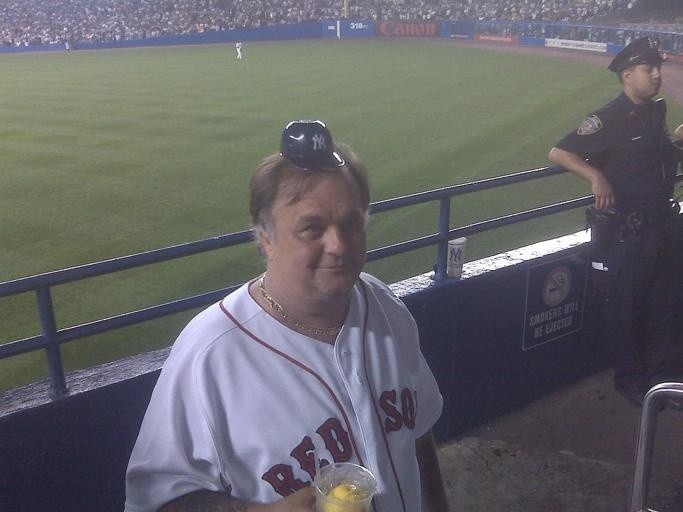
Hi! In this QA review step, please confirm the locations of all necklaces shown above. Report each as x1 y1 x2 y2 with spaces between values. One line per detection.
258 273 353 337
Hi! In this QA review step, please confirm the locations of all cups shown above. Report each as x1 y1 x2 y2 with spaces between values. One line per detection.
446 237 468 269
314 463 378 511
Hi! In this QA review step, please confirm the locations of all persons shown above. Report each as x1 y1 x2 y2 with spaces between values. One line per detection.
122 117 452 512
0 1 683 60
546 37 682 412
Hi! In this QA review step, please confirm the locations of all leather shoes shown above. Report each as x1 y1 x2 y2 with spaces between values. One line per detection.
614 385 683 411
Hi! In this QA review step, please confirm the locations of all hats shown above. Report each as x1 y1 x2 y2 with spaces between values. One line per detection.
280 120 346 170
608 36 664 73
